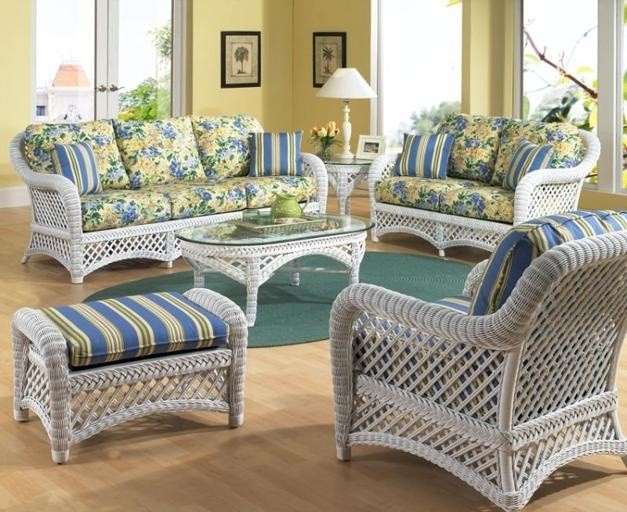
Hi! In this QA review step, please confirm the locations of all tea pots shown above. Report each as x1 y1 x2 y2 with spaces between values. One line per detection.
269 191 309 218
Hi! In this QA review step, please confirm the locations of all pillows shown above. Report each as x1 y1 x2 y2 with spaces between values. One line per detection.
434 111 507 184
488 120 584 187
467 209 626 316
23 114 304 197
397 131 458 181
502 138 555 192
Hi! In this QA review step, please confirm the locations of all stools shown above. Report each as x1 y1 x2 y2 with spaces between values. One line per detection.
12 287 248 465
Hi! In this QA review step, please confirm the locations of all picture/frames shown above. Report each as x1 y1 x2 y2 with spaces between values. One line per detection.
313 32 346 87
356 135 386 160
220 31 261 88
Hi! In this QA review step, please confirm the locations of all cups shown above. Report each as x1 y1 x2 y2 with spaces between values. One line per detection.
241 209 260 226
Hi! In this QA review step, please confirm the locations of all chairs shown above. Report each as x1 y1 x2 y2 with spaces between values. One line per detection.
329 210 627 512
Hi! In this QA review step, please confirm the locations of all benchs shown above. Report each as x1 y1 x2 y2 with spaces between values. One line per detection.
8 120 328 284
367 111 601 258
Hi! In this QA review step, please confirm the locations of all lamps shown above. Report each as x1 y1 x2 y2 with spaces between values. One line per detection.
315 67 377 159
51 64 93 120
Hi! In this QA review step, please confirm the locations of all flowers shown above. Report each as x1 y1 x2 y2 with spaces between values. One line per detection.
309 121 345 150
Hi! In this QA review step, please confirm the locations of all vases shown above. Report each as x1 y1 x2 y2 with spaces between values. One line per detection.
315 146 331 162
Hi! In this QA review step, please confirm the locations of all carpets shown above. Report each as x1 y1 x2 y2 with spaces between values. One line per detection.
82 251 477 348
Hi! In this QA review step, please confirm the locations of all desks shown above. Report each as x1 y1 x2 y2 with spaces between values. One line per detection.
325 158 375 215
